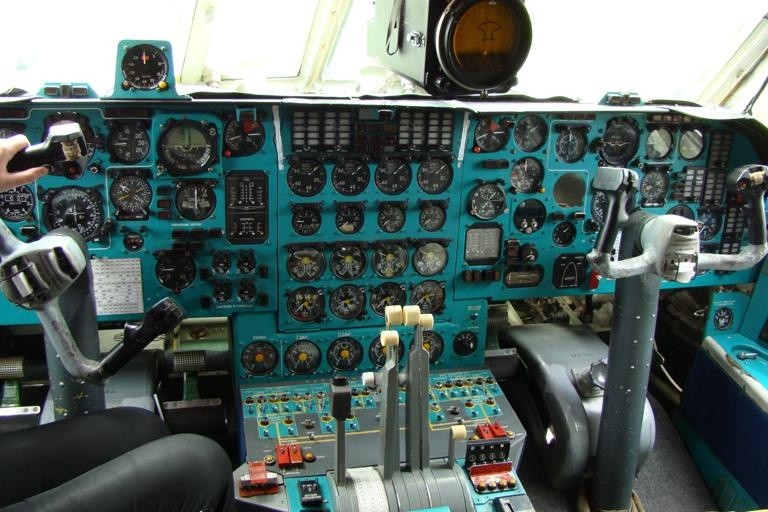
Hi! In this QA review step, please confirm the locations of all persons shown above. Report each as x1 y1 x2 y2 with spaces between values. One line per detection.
0 135 235 512
